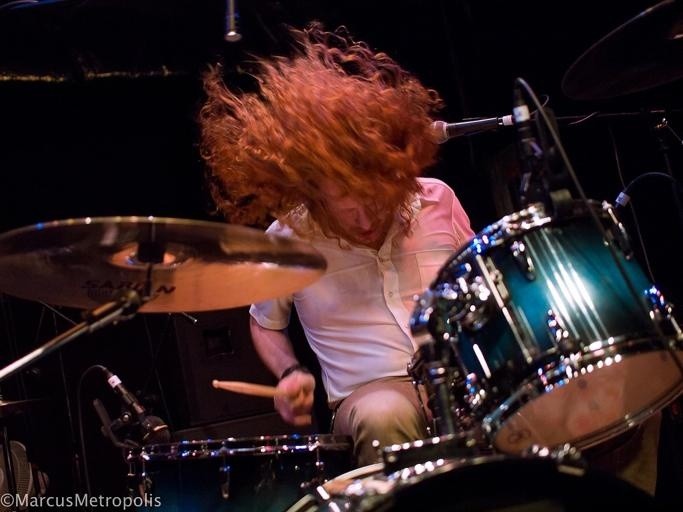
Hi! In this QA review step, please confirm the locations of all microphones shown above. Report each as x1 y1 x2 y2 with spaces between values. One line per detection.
426 115 513 144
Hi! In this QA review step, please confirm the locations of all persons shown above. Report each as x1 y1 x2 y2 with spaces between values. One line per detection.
202 23 484 464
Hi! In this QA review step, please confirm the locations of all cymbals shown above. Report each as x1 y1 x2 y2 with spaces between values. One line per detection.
0 214 330 314
561 3 683 101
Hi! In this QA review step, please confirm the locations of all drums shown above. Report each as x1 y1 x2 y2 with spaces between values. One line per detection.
287 428 666 512
407 196 683 455
120 430 356 512
